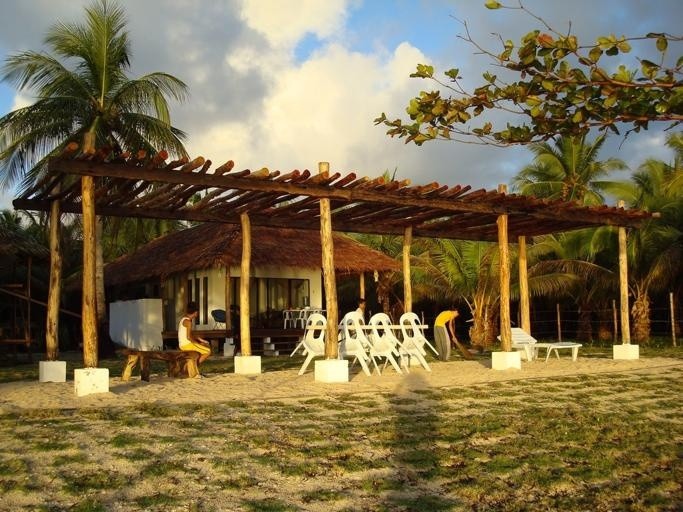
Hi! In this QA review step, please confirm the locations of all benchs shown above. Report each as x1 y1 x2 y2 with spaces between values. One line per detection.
121 350 201 383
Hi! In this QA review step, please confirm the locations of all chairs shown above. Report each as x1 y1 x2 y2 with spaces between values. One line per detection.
211 309 226 330
497 328 583 363
283 309 440 377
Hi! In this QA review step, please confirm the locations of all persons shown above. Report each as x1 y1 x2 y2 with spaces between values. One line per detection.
349 297 367 337
432 306 461 362
178 301 212 373
